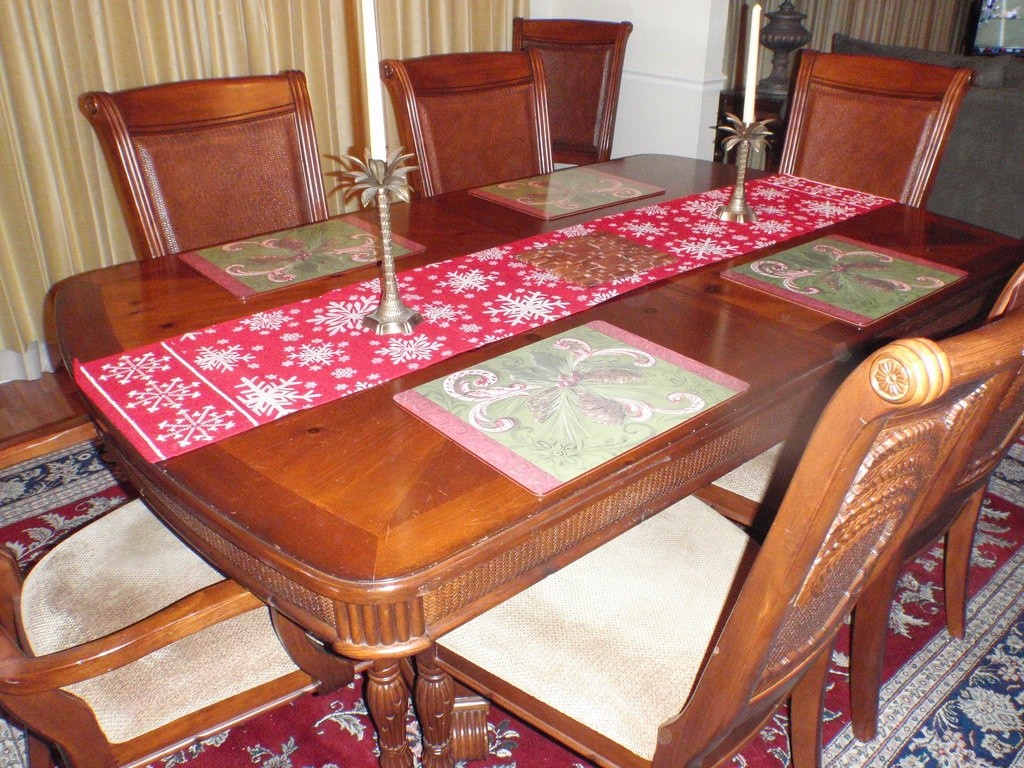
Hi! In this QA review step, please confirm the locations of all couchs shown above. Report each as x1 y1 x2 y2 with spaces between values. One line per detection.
831 32 1024 239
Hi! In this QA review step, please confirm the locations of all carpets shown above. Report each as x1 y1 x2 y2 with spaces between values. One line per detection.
0 439 1024 768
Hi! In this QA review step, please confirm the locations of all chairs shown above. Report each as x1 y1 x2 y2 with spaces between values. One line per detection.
380 47 553 198
77 70 329 259
688 262 1024 637
411 309 1024 768
513 16 633 165
0 413 372 768
778 49 980 209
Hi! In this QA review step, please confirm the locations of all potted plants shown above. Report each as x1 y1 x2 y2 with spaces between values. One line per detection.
711 113 774 225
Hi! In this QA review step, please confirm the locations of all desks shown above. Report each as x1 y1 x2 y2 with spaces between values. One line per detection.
41 154 1024 768
714 89 790 161
